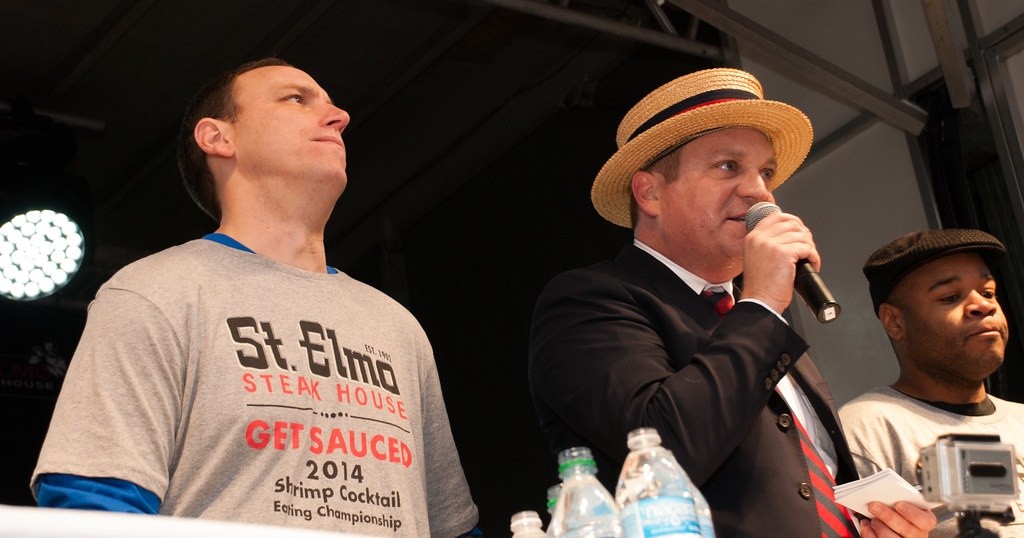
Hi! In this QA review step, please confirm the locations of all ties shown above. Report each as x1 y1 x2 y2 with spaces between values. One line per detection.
703 288 862 538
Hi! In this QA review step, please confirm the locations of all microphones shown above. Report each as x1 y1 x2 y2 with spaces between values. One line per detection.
745 201 841 324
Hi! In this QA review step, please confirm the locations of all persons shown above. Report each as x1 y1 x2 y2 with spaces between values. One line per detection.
528 68 937 538
28 56 485 538
837 230 1024 538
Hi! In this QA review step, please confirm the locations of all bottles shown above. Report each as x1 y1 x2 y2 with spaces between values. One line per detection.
510 511 549 538
667 449 715 538
615 427 701 538
552 448 623 538
546 483 562 538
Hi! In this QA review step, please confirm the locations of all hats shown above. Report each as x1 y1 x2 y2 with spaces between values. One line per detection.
863 227 1008 293
590 67 816 229
